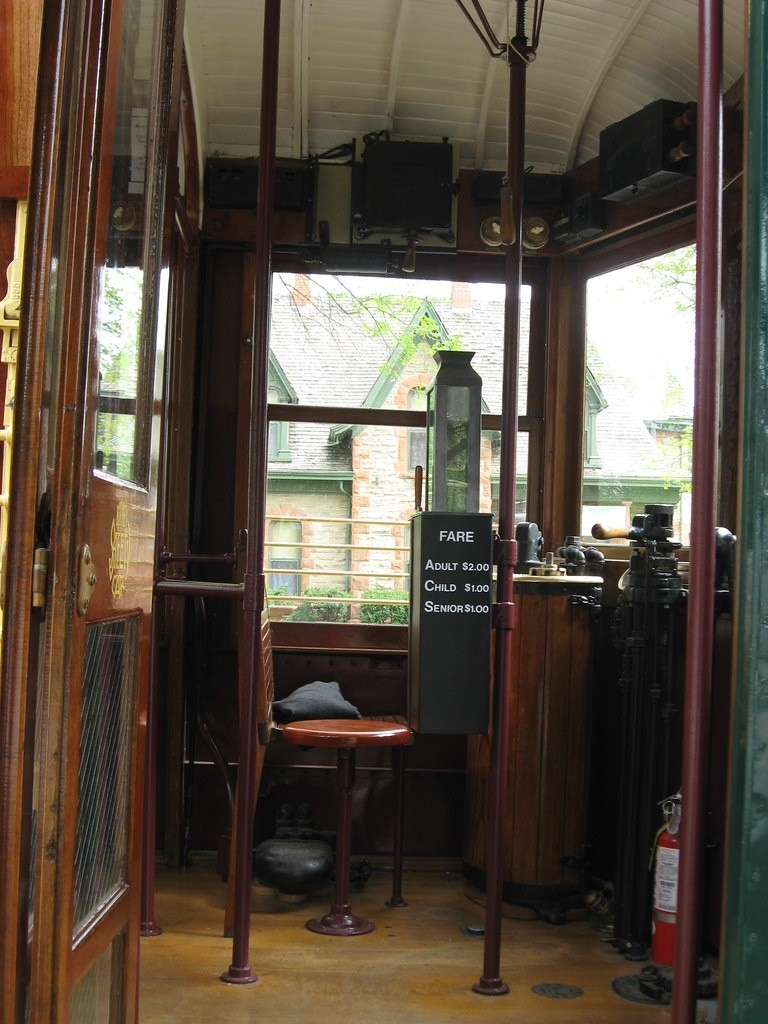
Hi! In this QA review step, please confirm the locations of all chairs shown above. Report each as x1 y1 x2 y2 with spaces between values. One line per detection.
222 583 414 943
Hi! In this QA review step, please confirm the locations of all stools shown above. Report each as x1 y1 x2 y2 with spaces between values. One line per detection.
281 720 411 940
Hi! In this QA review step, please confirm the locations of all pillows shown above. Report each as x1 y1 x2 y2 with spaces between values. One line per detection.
270 681 363 724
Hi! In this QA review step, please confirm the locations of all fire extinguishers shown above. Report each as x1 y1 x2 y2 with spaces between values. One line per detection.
648 789 683 970
